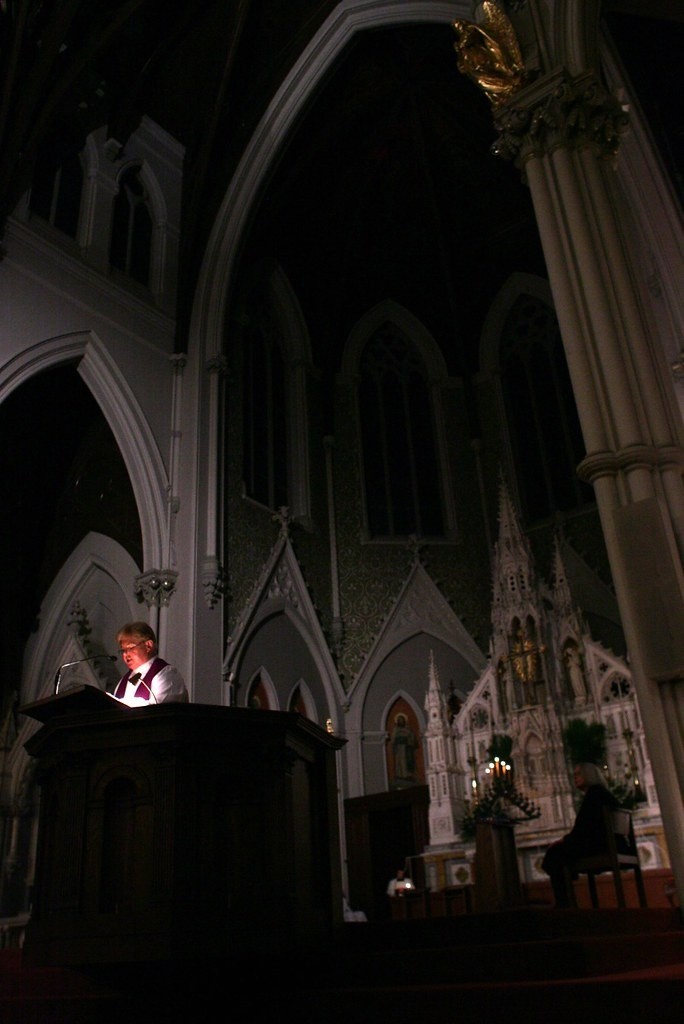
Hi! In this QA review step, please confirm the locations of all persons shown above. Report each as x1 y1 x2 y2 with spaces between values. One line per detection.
114 621 186 707
541 763 625 913
387 868 415 898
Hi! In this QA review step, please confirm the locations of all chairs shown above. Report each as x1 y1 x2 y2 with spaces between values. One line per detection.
560 808 648 910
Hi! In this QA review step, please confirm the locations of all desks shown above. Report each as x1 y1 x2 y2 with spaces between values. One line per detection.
401 888 431 919
442 882 473 915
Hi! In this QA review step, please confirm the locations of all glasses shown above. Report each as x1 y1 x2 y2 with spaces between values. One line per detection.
118 640 146 655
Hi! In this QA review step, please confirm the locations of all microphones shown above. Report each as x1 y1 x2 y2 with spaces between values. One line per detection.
53 655 118 695
